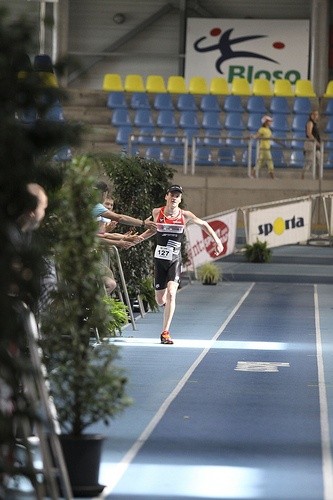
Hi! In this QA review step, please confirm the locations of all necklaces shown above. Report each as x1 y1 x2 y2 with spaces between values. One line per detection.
161 208 182 224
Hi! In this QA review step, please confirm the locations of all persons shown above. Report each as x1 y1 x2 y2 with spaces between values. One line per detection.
250 116 275 178
1 51 159 493
301 109 321 179
122 184 224 344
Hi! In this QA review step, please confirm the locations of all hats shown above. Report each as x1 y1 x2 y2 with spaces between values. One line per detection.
260 115 272 123
166 184 183 195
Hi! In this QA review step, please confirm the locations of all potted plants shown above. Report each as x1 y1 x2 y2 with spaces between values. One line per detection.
136 273 160 312
196 260 223 285
33 153 133 498
242 236 273 263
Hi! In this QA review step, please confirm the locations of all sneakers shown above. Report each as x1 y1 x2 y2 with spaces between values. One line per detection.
160 331 173 344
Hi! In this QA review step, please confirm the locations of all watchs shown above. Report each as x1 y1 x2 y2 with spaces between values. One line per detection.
141 220 145 229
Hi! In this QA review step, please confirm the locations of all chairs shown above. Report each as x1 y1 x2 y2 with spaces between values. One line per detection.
14 53 333 169
